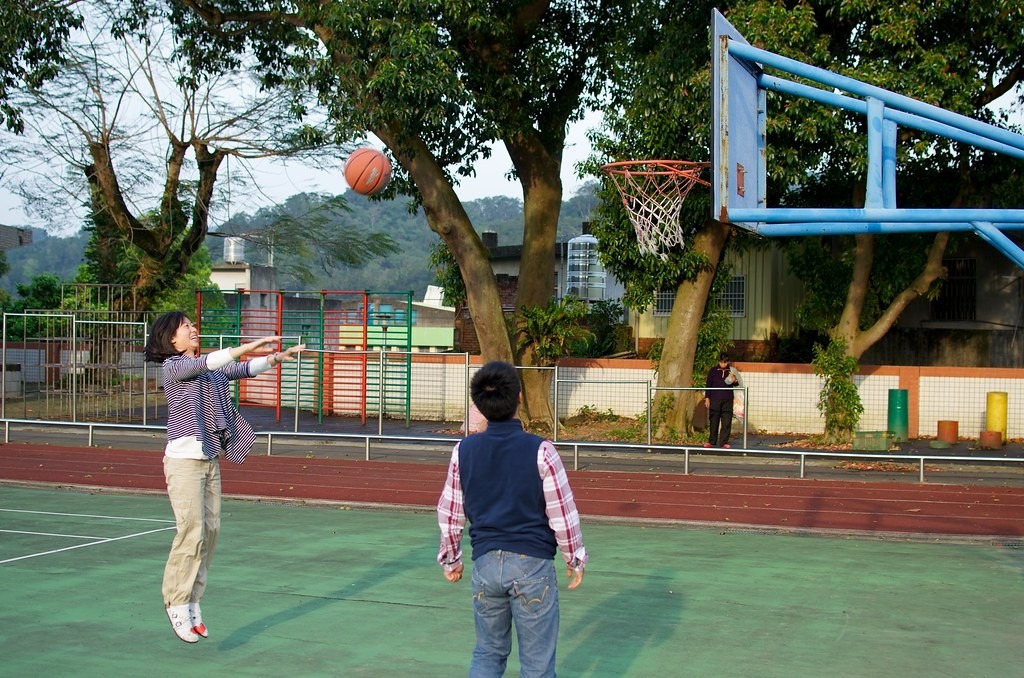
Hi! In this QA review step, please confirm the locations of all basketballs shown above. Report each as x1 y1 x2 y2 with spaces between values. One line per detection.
344 148 391 196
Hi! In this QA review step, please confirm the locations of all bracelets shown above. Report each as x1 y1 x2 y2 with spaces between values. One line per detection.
274 354 281 363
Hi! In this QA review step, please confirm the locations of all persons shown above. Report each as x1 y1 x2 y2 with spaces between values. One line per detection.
142 312 306 643
437 362 588 678
704 352 740 448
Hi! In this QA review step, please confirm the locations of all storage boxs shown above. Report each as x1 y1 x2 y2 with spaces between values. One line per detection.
853 430 896 451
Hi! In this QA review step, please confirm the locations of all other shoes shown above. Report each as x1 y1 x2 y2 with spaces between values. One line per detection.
703 443 716 448
190 603 208 638
166 603 199 644
721 444 731 449
